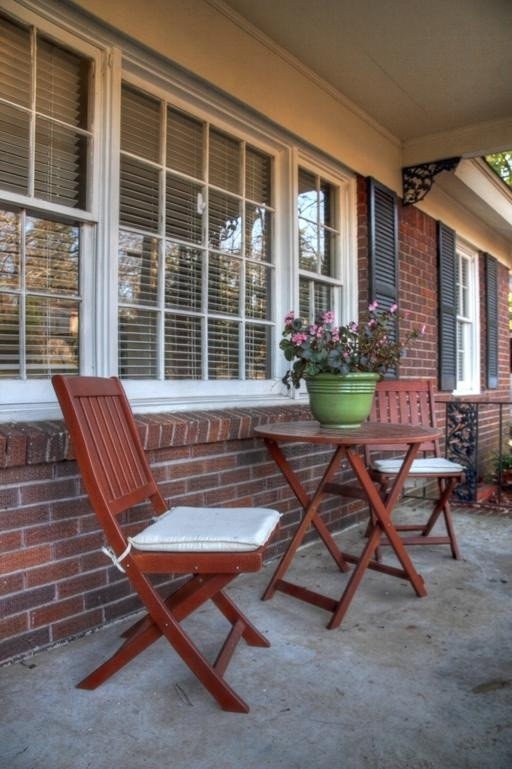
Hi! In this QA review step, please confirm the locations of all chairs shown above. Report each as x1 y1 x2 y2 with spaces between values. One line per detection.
362 378 469 565
49 373 284 714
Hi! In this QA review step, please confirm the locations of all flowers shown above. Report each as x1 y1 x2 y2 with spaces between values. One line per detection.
276 297 429 389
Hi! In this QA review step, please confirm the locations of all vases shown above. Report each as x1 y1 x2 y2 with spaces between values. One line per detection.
298 373 383 429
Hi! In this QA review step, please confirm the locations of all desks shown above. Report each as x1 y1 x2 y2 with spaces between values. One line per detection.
254 421 444 630
